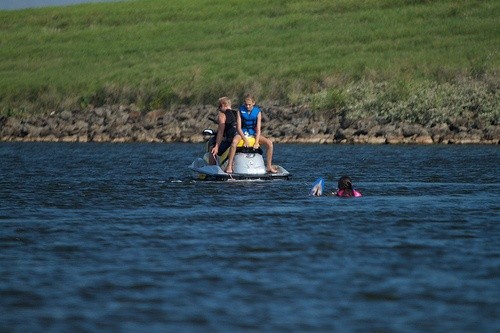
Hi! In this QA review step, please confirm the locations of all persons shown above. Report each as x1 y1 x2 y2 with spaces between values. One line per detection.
335 176 363 197
210 96 238 165
225 94 277 174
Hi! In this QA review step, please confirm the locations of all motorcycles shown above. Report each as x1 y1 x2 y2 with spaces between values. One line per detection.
183 116 290 185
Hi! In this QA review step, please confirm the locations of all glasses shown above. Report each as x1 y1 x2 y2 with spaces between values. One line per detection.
218 106 220 109
245 103 252 106
